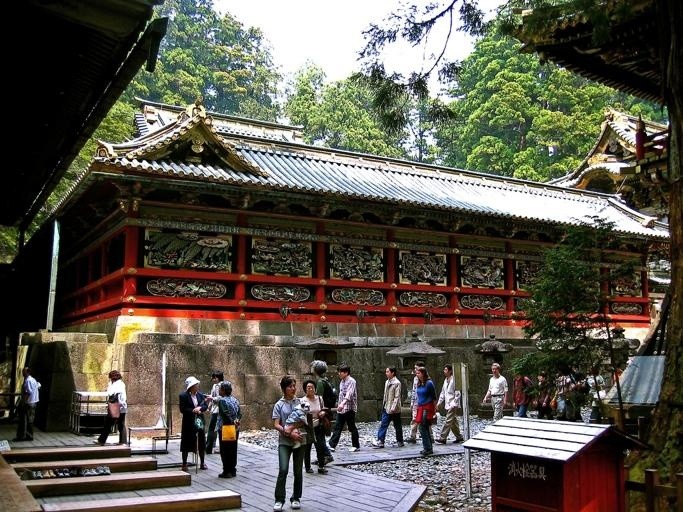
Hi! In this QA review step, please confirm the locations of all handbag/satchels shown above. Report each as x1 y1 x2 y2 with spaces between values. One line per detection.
322 415 331 436
222 425 236 441
323 384 336 408
536 403 552 415
109 402 121 418
550 399 557 409
195 414 204 430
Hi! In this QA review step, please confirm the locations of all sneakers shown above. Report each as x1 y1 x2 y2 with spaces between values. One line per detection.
292 500 300 509
32 466 111 479
307 443 335 473
274 502 282 512
349 446 359 452
420 447 433 455
435 440 446 445
201 463 207 469
93 440 105 445
372 437 416 447
219 472 235 478
182 463 188 471
453 440 464 443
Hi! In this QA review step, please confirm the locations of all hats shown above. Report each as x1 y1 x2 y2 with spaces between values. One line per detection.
184 376 200 392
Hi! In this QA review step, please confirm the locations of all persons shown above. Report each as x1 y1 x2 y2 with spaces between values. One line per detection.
512 366 623 423
12 367 39 443
204 371 224 454
272 360 464 512
179 376 208 472
92 370 127 446
482 362 509 424
214 380 241 478
